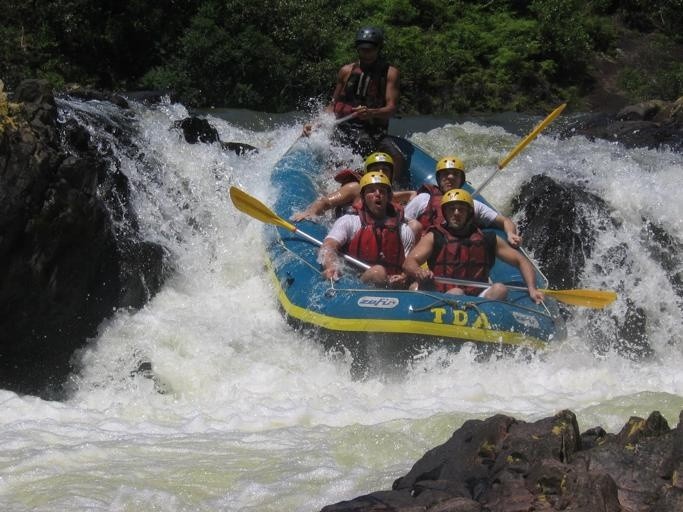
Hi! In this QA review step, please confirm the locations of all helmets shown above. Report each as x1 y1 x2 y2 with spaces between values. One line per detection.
440 189 473 208
436 157 465 174
360 172 392 194
365 153 393 169
355 26 383 45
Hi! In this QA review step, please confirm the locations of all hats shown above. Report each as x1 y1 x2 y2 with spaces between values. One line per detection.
357 43 375 49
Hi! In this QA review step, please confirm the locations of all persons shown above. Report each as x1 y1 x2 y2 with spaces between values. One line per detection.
300 25 408 178
400 187 545 306
286 150 418 226
316 169 428 292
400 155 524 249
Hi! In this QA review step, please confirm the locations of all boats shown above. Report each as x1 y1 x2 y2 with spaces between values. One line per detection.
260 119 565 365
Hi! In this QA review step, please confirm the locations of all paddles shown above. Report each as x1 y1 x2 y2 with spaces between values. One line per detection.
229 186 374 270
469 104 568 198
432 276 618 308
